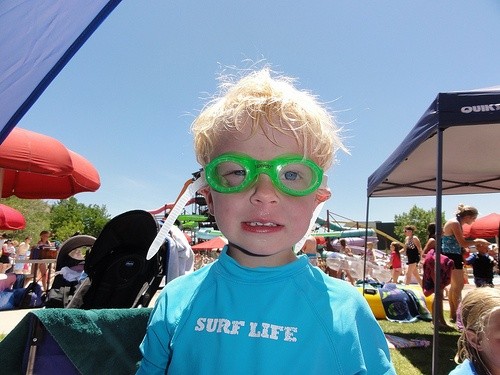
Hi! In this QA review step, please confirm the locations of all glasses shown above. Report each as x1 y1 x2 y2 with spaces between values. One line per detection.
202 152 328 197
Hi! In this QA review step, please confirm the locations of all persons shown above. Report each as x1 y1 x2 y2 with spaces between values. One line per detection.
448 283 500 375
441 203 482 322
0 237 31 274
422 223 435 259
399 225 423 285
32 231 52 292
135 49 398 375
383 241 402 283
463 238 498 288
324 237 376 286
302 235 317 265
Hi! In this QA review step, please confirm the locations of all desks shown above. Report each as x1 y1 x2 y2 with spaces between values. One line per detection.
14 259 57 294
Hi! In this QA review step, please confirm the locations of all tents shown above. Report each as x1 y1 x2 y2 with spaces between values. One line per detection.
358 87 500 375
191 234 229 271
460 212 500 241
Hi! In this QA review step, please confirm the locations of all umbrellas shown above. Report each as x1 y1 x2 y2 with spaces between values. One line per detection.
1 127 100 230
